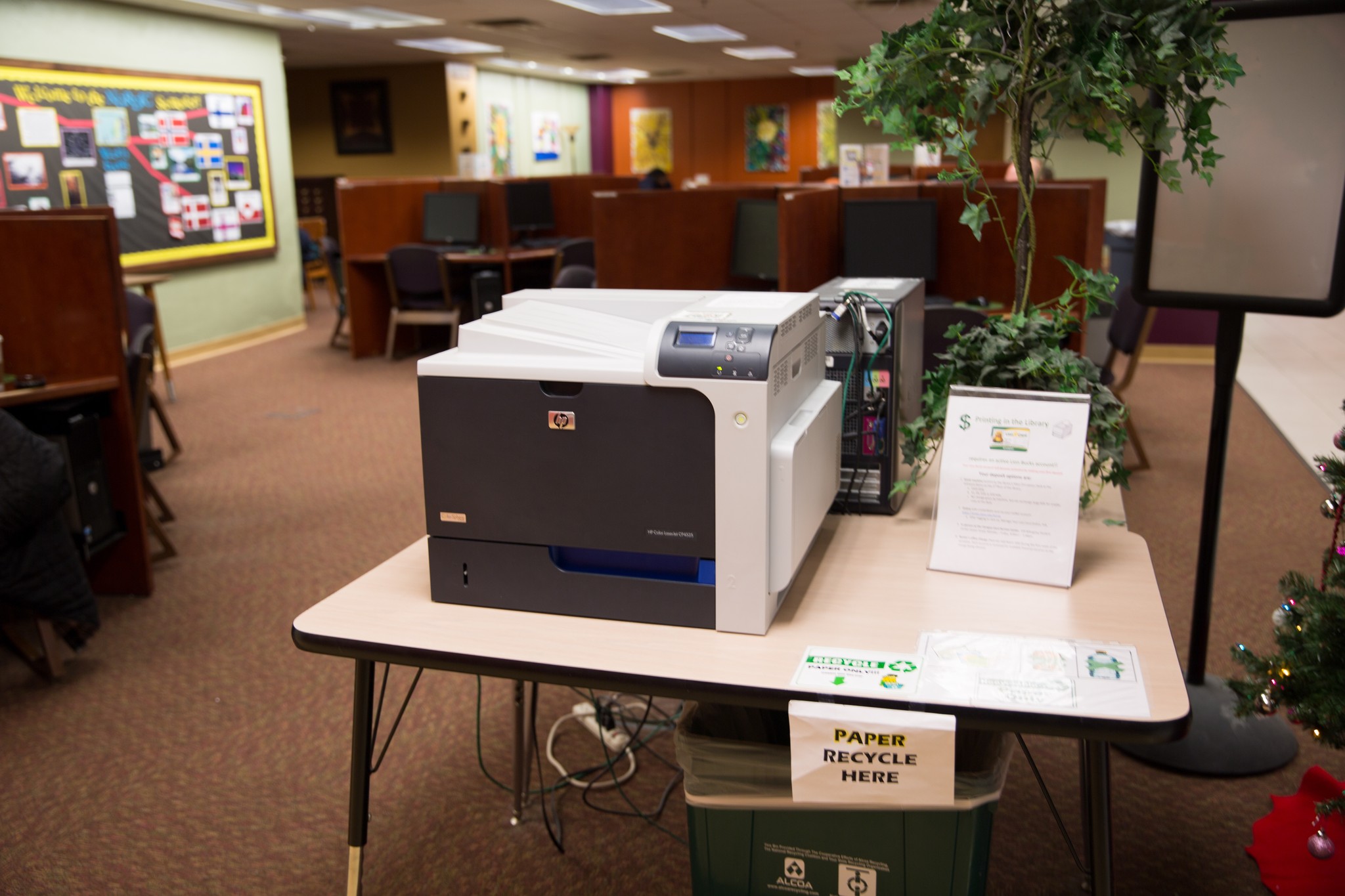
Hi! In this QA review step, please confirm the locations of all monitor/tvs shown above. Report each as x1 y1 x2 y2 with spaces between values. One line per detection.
841 199 939 283
420 194 483 243
505 181 555 231
730 199 781 281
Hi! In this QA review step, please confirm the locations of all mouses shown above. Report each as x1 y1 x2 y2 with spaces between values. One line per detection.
477 245 488 255
966 294 990 307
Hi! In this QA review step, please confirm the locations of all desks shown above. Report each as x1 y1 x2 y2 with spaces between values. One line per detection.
294 498 1196 896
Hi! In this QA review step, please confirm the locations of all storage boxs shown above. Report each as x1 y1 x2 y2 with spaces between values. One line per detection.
677 699 1021 896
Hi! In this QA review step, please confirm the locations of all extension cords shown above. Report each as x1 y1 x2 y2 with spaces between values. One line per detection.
573 702 632 751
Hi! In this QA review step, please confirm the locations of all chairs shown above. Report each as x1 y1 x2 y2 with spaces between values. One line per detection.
2 257 187 672
352 184 596 364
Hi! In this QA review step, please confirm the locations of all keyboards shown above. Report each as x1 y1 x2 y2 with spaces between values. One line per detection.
406 243 468 254
520 236 571 250
920 296 955 305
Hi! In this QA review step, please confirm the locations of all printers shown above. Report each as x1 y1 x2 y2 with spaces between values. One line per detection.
418 285 843 636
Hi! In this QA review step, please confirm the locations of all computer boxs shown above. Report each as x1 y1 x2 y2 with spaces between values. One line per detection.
809 274 927 514
